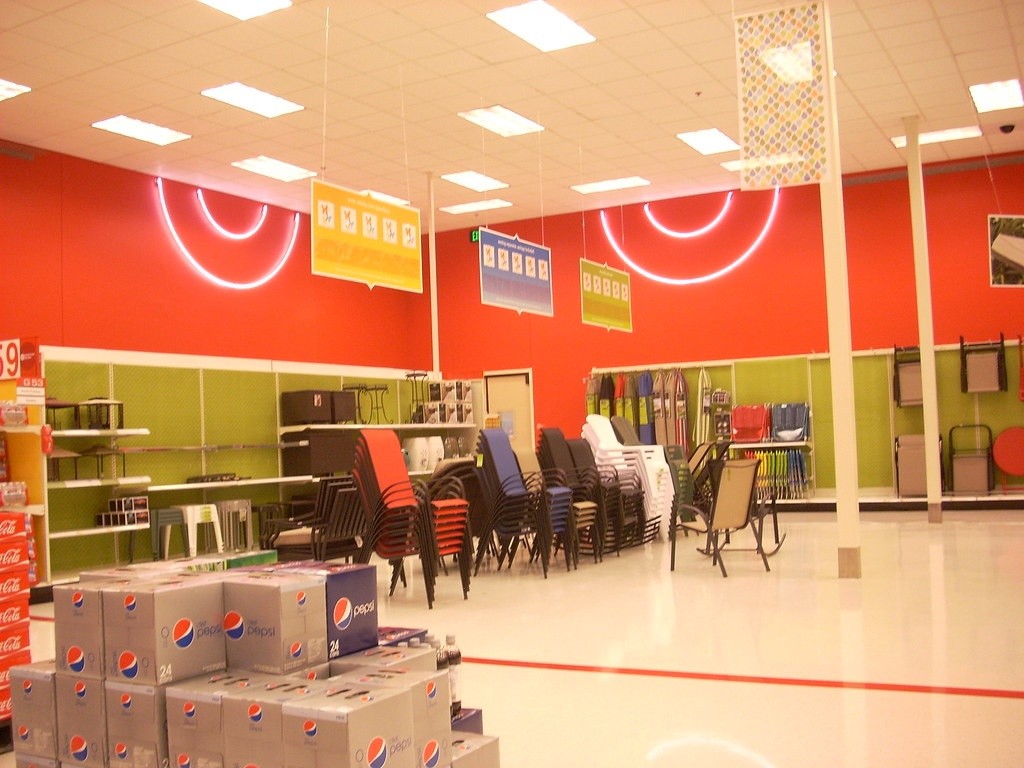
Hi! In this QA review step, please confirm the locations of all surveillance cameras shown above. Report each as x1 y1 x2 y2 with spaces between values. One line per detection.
1000 125 1015 134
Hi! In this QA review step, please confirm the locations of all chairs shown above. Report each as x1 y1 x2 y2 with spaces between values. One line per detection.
732 402 771 443
696 460 787 567
670 458 771 578
959 332 1008 393
895 434 946 499
258 413 733 611
893 343 923 408
948 424 995 492
770 402 810 442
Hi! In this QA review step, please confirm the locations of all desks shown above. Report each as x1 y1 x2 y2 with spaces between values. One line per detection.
993 427 1024 497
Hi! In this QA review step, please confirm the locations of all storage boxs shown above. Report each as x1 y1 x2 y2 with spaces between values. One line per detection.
223 570 328 676
282 390 358 426
0 646 32 691
0 532 29 572
419 380 474 424
79 567 170 586
102 578 226 688
0 617 30 660
0 686 12 721
53 578 144 681
9 626 500 768
0 560 30 605
282 431 354 476
0 512 25 538
0 599 30 633
247 558 378 661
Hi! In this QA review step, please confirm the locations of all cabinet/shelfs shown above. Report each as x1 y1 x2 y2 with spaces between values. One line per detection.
271 423 479 545
138 475 313 573
0 423 151 589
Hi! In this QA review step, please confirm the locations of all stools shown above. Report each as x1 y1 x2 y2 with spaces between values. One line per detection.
128 498 254 564
